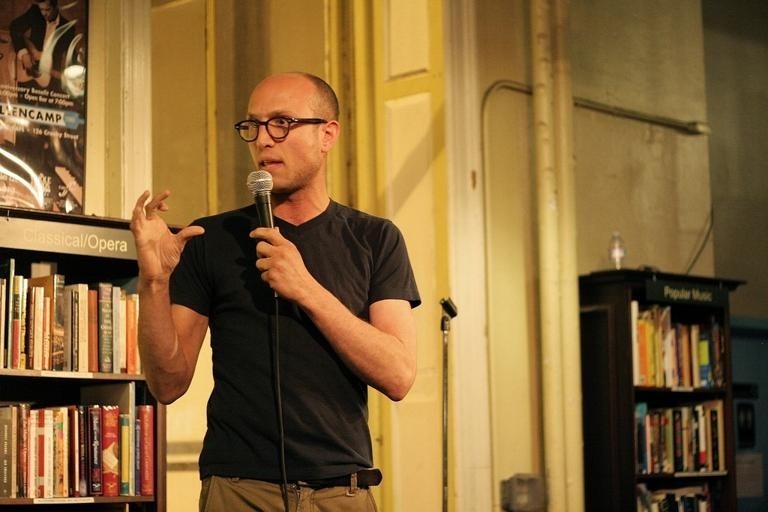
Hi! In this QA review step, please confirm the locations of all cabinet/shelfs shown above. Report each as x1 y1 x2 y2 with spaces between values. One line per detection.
0 204 173 512
578 266 750 512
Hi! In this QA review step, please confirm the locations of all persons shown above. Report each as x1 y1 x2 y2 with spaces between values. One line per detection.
8 1 79 176
129 70 424 511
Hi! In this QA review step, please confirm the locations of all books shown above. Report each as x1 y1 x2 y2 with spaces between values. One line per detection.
1 402 154 497
1 260 141 375
629 293 732 510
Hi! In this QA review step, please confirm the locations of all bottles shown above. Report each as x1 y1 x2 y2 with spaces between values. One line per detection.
608 231 628 271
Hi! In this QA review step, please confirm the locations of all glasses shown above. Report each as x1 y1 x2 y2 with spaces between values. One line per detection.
234 115 328 142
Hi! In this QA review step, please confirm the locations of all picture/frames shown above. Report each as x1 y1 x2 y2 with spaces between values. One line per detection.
0 0 90 213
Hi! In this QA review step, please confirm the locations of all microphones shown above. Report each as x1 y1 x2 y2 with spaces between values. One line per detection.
246 169 279 298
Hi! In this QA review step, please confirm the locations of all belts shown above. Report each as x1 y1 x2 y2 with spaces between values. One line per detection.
298 469 382 485
